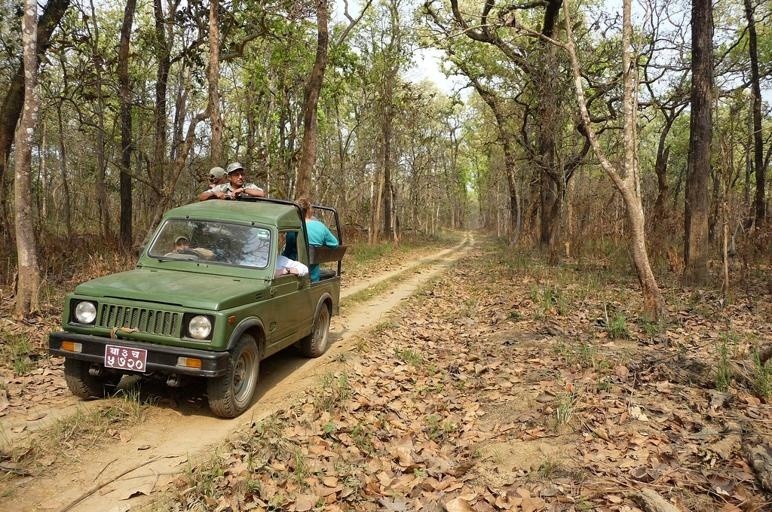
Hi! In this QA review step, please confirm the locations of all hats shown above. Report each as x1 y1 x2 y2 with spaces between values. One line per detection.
174 232 189 244
209 167 227 183
227 163 243 173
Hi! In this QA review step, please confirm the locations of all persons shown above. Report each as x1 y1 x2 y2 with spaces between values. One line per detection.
163 162 339 283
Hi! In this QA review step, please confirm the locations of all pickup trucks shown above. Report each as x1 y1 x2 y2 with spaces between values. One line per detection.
48 197 345 417
